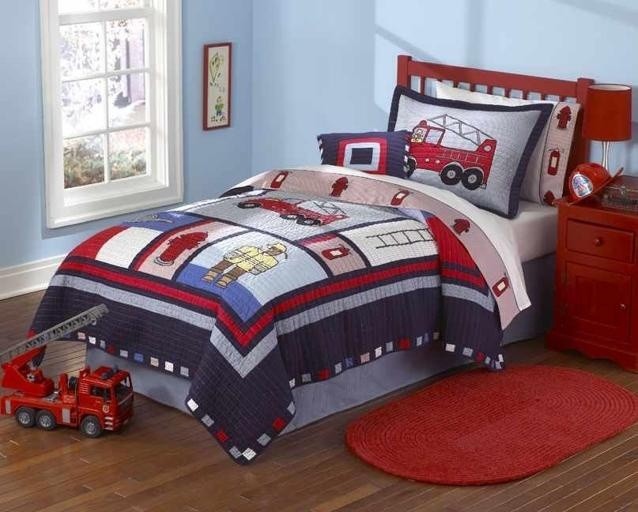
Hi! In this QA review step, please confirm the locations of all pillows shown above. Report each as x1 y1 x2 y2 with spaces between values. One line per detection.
315 80 583 219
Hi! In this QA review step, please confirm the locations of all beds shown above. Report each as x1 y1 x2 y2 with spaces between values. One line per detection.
65 55 597 442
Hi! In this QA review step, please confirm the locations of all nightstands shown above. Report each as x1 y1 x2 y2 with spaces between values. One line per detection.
550 178 638 375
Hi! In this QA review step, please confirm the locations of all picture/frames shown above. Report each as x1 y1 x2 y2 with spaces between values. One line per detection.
202 41 230 131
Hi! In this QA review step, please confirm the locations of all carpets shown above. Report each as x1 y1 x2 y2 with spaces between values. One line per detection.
343 360 637 487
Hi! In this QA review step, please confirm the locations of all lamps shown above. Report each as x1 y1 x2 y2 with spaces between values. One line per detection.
581 82 631 175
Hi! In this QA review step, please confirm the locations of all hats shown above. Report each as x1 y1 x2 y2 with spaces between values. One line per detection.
567 158 624 206
